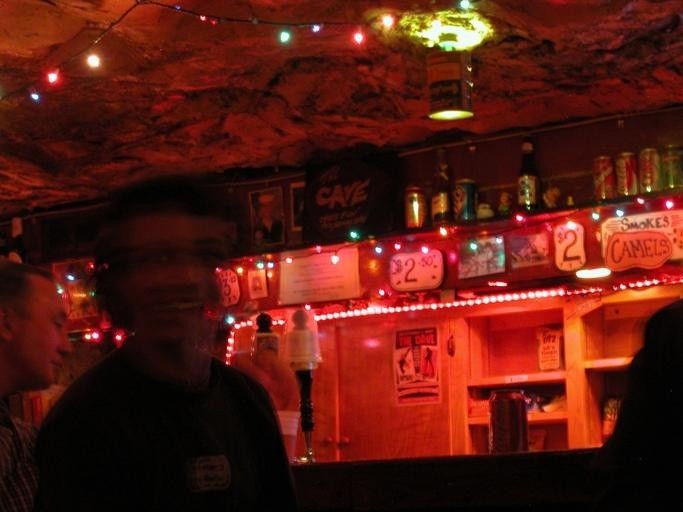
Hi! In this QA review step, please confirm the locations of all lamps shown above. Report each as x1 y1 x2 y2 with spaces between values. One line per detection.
426 47 474 122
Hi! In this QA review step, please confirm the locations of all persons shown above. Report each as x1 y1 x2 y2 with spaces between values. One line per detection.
0 259 83 509
601 294 682 510
34 182 282 510
291 306 322 467
251 312 283 368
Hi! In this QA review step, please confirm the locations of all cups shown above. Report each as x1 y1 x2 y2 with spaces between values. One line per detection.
281 308 325 363
274 410 301 463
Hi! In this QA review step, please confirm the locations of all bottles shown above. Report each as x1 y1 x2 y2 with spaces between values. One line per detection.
433 144 453 228
514 133 539 212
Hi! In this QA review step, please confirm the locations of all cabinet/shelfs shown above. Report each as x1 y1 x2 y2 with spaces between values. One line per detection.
446 302 575 455
268 309 446 466
576 284 683 450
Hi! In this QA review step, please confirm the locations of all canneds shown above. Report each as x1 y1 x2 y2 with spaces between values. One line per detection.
430 183 451 228
488 388 528 454
404 184 430 230
451 178 478 225
637 147 663 195
661 143 683 192
591 155 617 201
615 152 639 197
